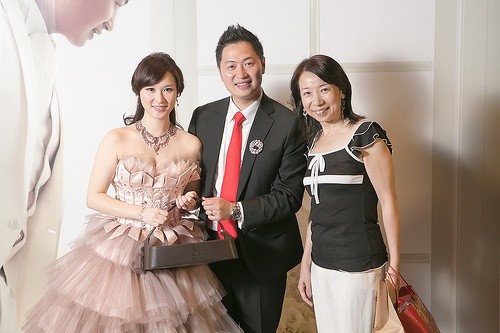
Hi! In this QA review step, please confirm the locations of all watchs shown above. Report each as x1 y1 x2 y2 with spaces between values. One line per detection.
229 203 242 221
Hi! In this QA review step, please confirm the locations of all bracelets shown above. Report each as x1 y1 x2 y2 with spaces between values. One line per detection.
139 206 147 222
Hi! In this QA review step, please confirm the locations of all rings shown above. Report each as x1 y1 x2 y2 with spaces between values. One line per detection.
182 207 187 209
210 211 214 217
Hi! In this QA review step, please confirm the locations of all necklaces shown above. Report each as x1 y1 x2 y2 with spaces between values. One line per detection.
136 120 178 155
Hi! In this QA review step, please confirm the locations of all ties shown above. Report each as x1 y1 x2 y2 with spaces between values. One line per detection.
217 112 246 240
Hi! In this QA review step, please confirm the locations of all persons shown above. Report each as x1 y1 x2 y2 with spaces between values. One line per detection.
0 0 131 333
187 25 308 333
21 53 246 333
290 54 400 333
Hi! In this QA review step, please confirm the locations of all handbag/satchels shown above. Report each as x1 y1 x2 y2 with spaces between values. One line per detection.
387 265 441 333
372 275 405 333
